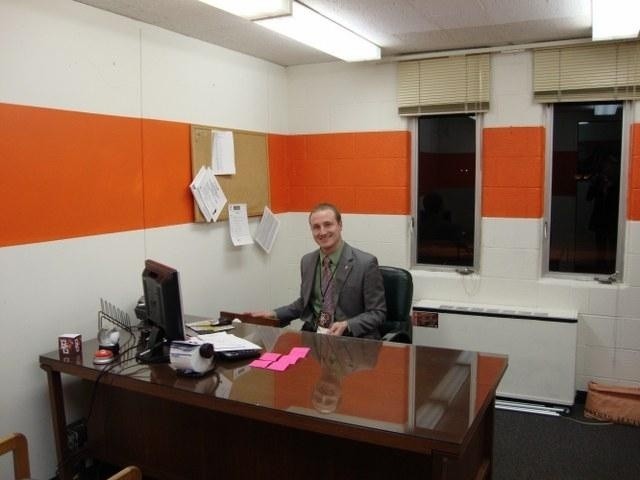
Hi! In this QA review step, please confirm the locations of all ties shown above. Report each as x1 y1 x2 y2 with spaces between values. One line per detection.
318 257 335 330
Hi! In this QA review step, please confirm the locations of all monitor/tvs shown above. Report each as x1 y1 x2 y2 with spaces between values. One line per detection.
135 259 185 365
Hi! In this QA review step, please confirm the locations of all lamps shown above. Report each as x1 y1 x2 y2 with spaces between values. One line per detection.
195 0 383 65
588 0 640 43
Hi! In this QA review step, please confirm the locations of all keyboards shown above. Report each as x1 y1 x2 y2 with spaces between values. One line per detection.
218 350 260 361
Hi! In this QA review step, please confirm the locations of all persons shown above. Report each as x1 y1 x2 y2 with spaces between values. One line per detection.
239 202 387 343
299 329 382 416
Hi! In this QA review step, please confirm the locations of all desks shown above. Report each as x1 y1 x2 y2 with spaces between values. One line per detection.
40 313 508 479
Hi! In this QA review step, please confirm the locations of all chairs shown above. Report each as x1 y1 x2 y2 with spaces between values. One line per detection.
300 264 413 346
